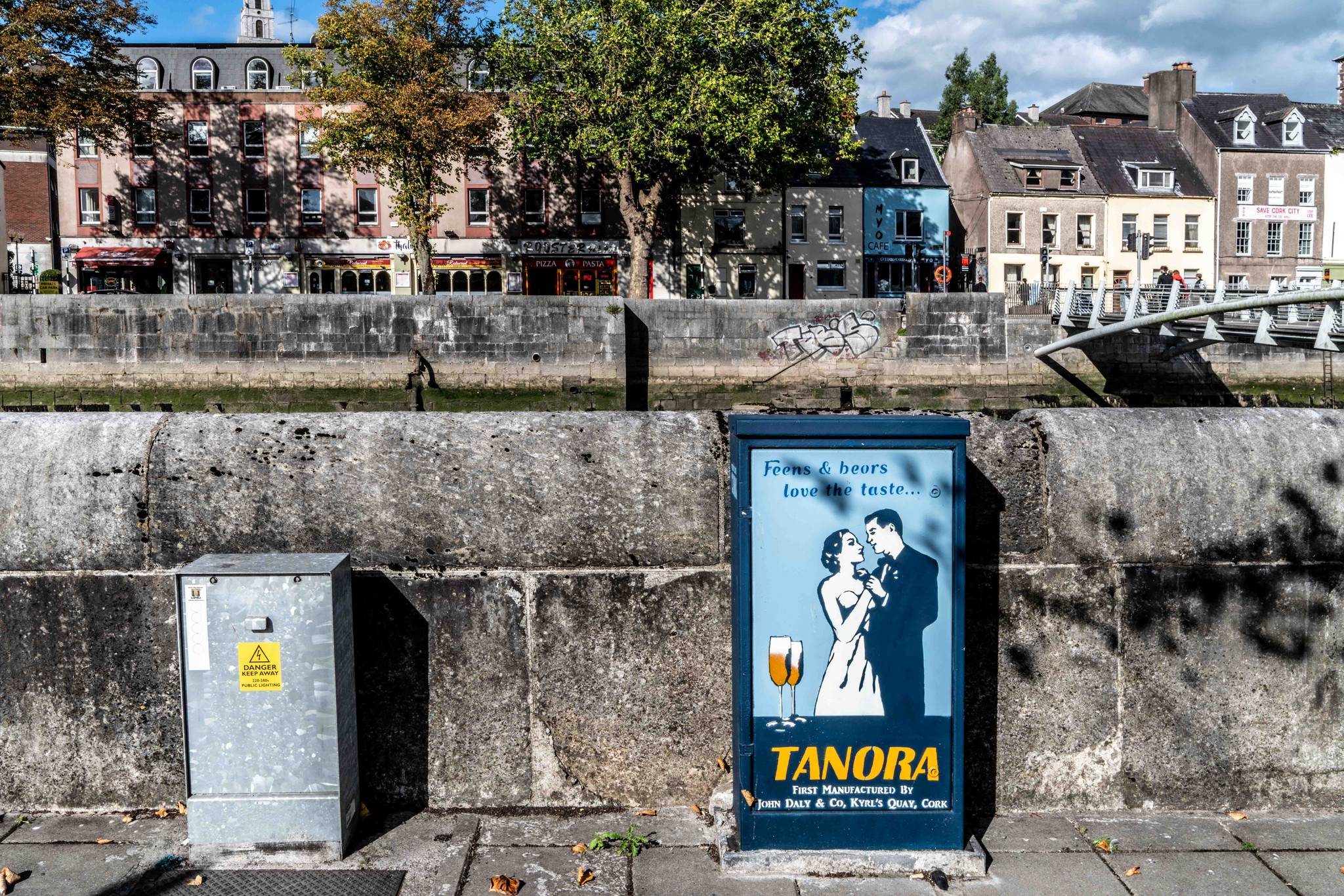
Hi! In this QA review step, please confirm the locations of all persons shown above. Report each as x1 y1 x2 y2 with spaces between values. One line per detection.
78 291 85 295
1016 278 1031 306
972 277 988 292
1194 273 1210 317
879 277 889 291
1154 265 1187 312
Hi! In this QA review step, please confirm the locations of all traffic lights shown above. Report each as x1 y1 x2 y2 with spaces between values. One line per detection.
1142 233 1154 260
1040 246 1052 262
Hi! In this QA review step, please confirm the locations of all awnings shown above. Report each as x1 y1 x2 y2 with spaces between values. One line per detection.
71 247 165 268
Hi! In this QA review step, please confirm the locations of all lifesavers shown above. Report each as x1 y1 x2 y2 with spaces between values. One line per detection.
934 266 952 283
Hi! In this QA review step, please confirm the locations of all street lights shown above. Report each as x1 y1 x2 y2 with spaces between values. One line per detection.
8 229 26 293
